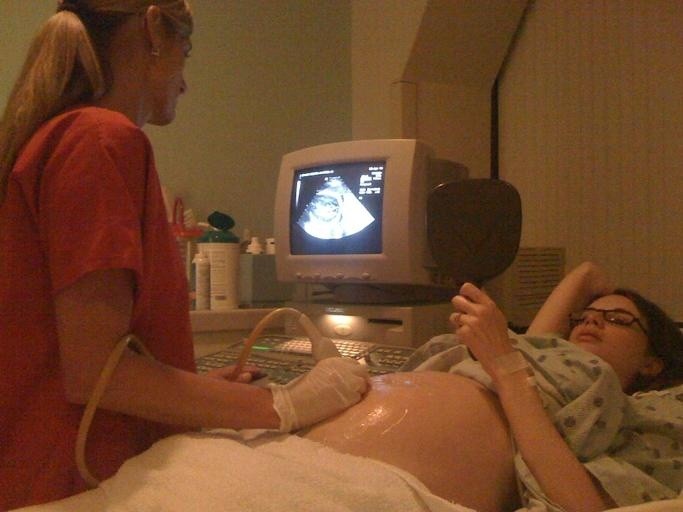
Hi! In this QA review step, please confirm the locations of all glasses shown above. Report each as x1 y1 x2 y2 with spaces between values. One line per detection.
569 307 659 356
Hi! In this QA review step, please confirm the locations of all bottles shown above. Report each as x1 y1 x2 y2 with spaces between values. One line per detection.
193 249 210 312
197 228 240 311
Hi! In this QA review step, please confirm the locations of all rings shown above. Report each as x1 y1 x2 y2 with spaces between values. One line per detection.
453 309 466 328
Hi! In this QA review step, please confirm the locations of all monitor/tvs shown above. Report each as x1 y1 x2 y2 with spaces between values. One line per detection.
273 138 470 305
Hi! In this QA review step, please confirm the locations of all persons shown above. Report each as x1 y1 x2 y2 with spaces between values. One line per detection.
0 0 372 512
1 259 683 511
